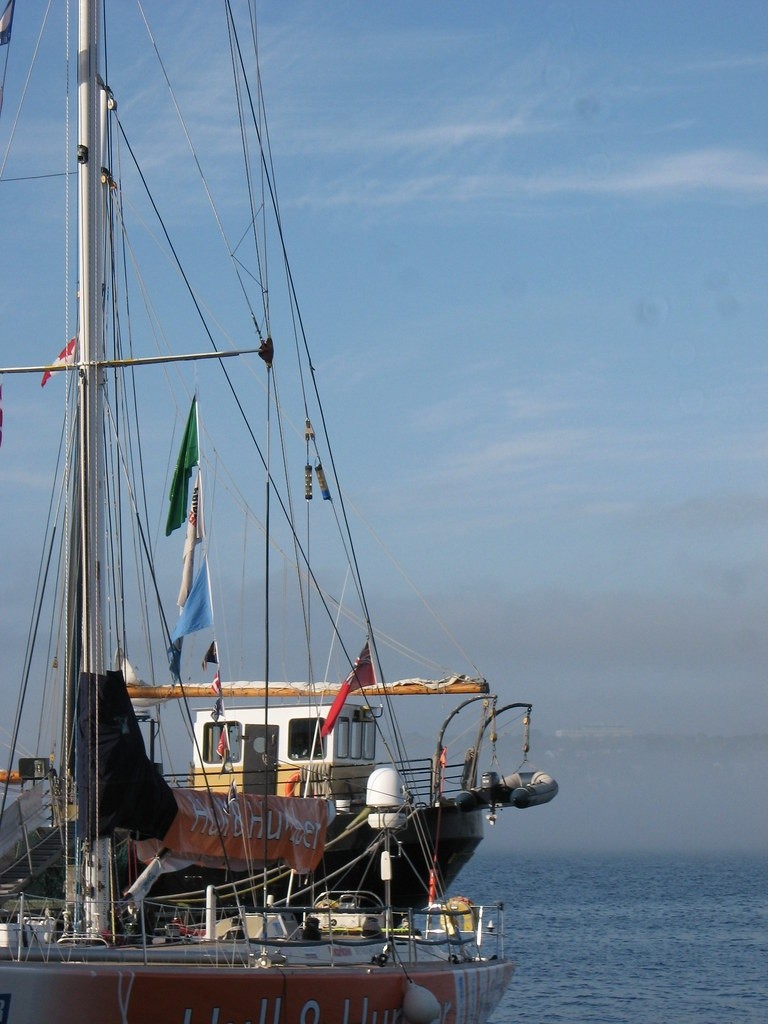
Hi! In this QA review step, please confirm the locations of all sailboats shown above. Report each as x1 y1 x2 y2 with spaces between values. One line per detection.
0 0 522 1024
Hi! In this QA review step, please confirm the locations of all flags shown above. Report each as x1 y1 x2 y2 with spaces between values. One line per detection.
165 396 238 816
40 338 78 387
316 642 377 738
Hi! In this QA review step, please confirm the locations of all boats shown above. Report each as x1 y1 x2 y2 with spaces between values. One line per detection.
0 673 557 928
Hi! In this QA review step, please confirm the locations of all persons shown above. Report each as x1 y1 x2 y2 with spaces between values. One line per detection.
402 917 423 936
123 898 157 944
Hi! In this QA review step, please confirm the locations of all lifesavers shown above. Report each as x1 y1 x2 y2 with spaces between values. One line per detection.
439 896 478 935
285 772 301 797
